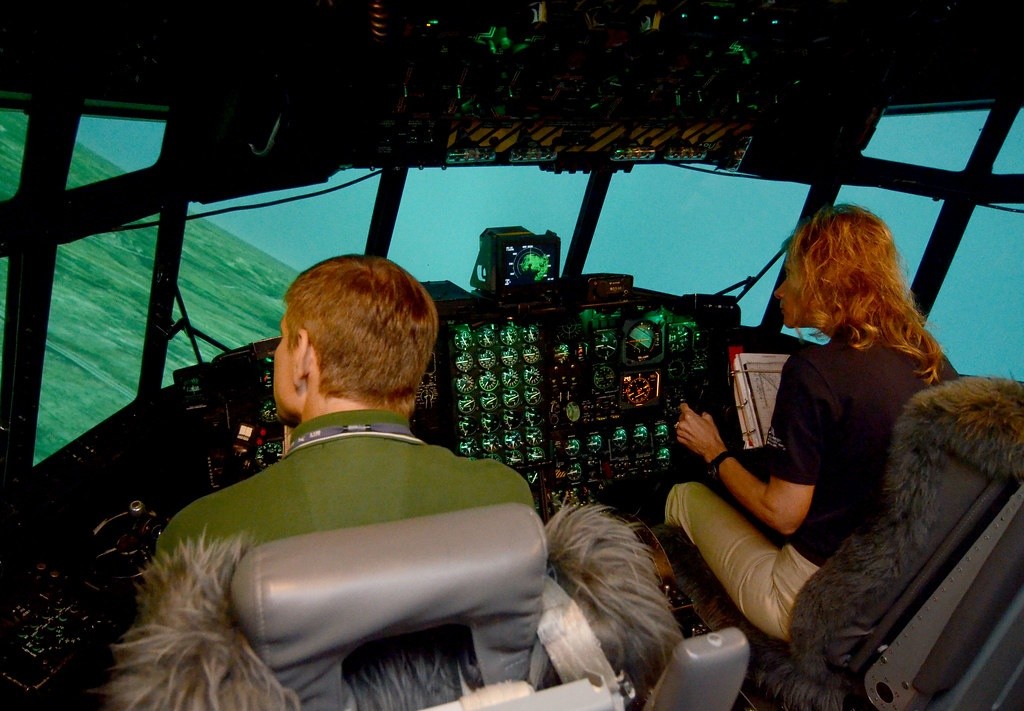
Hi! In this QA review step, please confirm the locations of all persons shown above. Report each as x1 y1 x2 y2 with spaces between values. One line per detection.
118 256 537 632
665 206 960 642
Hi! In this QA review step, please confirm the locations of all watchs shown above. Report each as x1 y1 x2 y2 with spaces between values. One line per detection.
706 452 734 481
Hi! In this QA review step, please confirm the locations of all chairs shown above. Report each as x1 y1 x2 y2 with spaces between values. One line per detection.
657 375 1023 711
231 501 748 711
